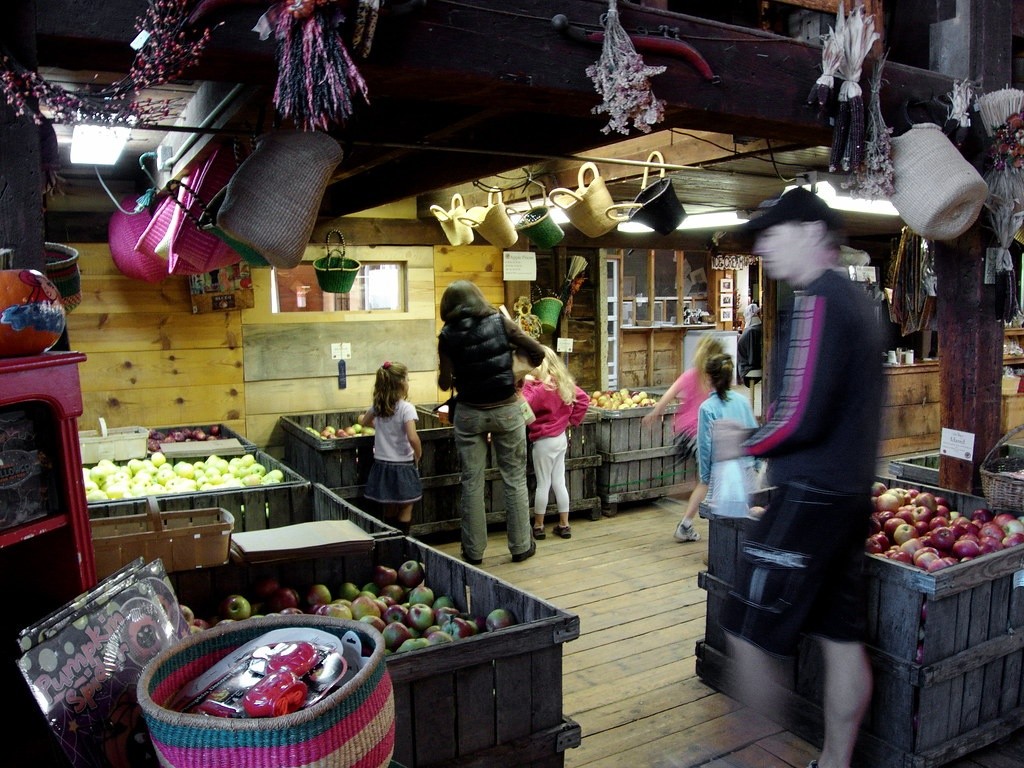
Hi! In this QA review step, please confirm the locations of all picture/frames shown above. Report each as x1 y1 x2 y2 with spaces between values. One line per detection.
720 279 733 292
720 294 733 308
720 309 733 322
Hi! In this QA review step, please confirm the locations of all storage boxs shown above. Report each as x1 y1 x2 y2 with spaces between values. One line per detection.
78 369 1024 768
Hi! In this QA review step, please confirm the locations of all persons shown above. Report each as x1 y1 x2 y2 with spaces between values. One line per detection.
362 361 423 537
437 279 545 566
521 345 589 538
710 186 889 768
697 354 762 565
641 335 725 542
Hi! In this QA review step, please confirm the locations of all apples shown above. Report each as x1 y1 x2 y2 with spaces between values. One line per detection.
178 559 514 653
147 425 221 450
79 451 283 504
306 414 375 440
587 389 657 410
865 480 1024 665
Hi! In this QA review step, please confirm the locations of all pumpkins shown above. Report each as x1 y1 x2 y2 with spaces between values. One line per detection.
0 269 67 357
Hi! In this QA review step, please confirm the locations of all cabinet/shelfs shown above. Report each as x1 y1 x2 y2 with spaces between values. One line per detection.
878 360 940 461
999 327 1024 441
1 352 98 597
